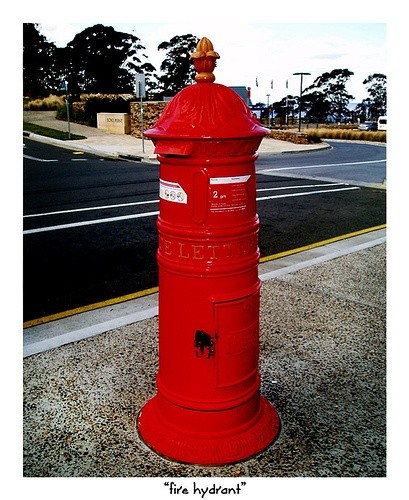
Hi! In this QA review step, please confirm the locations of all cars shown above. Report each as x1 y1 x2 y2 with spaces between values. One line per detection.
358 121 377 131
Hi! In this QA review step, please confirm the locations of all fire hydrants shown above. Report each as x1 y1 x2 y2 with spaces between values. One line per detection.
136 37 283 467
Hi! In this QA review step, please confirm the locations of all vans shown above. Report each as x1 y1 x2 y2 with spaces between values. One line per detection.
377 115 387 130
